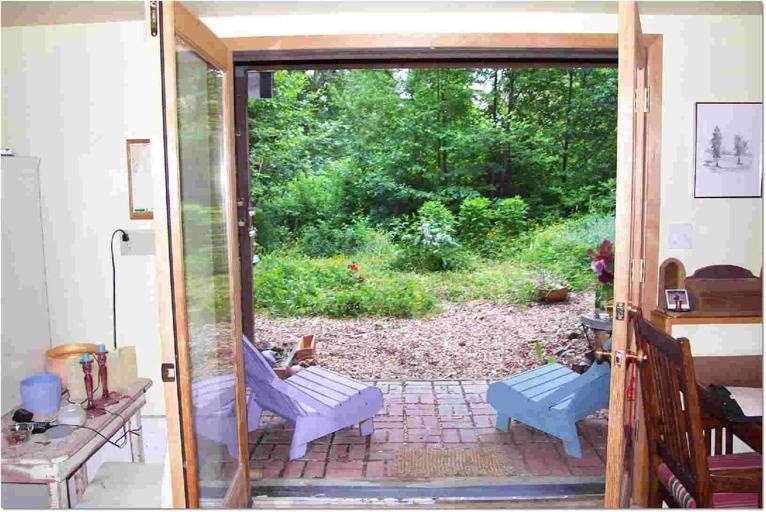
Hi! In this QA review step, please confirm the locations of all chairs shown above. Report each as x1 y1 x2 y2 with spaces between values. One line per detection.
242 332 383 461
191 372 239 460
631 310 763 511
487 337 611 460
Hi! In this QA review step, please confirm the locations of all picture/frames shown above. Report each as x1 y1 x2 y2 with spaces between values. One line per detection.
666 288 691 311
126 140 153 221
693 101 764 199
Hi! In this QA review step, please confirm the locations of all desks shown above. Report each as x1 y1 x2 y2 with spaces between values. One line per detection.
2 377 152 509
674 354 763 455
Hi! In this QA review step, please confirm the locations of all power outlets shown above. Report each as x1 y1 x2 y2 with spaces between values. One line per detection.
120 230 154 255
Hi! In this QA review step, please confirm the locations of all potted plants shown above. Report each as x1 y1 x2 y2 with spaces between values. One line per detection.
536 269 569 301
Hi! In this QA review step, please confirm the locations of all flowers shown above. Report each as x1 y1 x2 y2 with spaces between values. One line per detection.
588 240 615 283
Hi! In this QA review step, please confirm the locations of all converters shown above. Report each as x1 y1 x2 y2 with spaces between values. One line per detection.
12 408 34 423
16 421 50 435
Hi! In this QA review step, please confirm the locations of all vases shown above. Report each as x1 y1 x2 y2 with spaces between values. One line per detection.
597 283 613 309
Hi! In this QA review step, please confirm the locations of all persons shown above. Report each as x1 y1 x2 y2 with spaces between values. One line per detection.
672 292 683 311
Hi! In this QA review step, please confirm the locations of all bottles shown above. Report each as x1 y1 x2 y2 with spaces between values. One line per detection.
58 405 85 428
535 339 544 363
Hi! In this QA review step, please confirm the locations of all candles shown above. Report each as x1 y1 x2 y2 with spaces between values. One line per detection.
98 344 105 353
83 352 89 362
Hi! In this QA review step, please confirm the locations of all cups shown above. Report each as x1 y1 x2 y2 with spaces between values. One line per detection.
676 300 682 309
19 376 60 414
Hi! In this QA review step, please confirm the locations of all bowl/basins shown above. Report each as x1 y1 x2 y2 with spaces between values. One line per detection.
44 342 102 390
2 423 35 444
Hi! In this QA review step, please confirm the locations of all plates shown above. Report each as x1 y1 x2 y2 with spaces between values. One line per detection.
670 308 690 313
